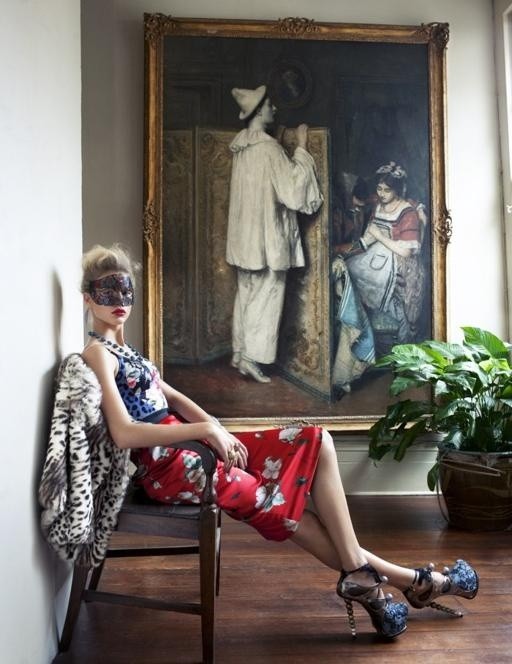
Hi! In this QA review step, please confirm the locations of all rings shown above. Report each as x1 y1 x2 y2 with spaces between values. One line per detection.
229 450 237 460
234 443 242 452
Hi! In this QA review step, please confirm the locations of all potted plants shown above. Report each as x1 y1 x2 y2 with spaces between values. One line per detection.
366 324 512 534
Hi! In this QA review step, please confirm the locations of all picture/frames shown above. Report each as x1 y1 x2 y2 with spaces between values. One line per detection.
142 8 451 432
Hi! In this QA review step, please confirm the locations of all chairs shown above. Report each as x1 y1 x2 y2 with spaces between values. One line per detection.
58 353 221 664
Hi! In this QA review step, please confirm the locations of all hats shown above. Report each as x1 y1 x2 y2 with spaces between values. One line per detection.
230 85 269 121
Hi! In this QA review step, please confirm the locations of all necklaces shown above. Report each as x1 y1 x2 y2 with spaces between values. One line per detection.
85 330 143 368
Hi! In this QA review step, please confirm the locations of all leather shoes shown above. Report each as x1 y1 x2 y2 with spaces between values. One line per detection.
237 358 271 384
230 352 242 369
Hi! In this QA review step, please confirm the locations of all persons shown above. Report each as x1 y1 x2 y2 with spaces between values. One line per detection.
328 170 372 265
82 244 479 638
331 162 430 395
227 85 324 385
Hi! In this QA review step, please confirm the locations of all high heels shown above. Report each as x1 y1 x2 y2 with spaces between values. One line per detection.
336 564 409 638
400 559 479 620
